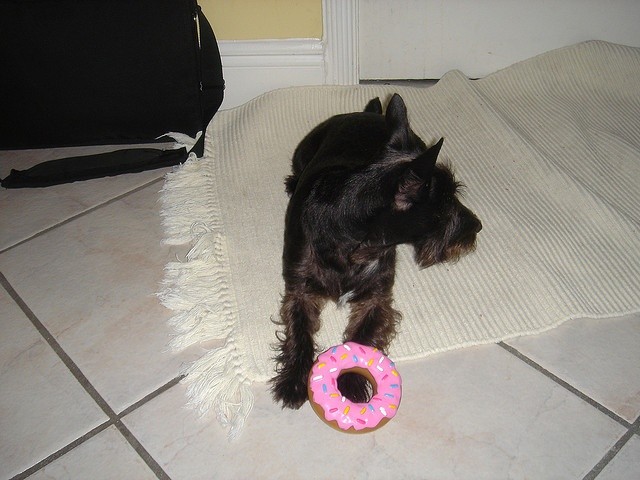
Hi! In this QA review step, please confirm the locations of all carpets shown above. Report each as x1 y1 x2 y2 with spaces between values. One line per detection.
157 41 640 438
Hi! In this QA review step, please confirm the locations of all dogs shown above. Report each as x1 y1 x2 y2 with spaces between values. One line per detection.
268 93 482 408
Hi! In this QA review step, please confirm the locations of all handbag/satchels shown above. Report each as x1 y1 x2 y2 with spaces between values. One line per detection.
0 0 226 189
